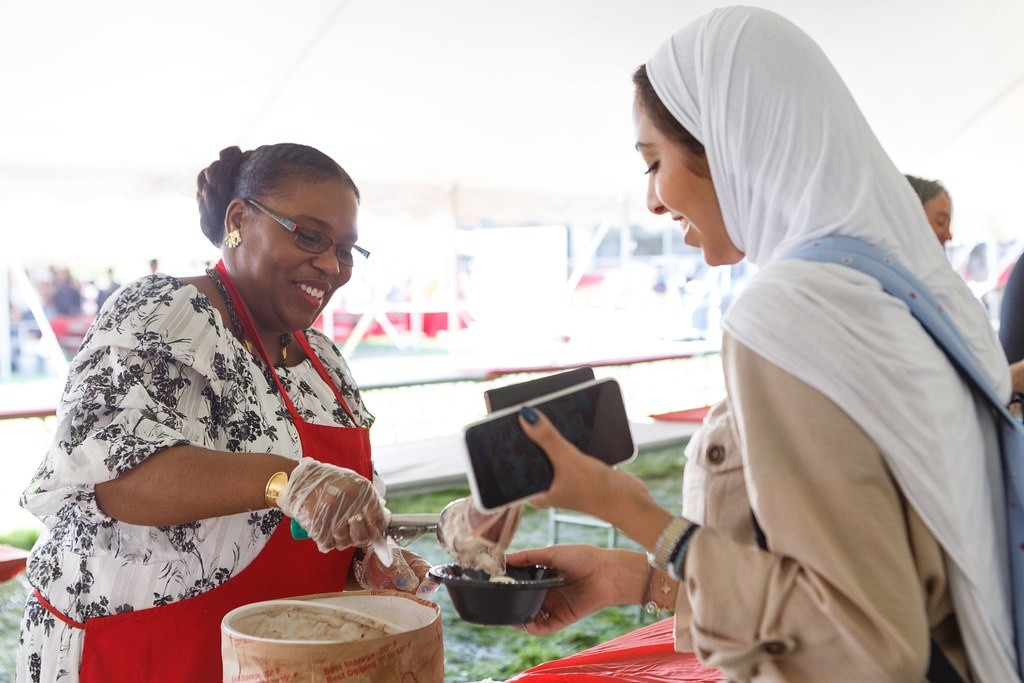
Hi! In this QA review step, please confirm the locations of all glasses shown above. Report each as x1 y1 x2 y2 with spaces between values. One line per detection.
240 194 370 268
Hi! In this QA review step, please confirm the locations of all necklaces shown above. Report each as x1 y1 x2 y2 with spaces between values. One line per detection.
206 269 291 372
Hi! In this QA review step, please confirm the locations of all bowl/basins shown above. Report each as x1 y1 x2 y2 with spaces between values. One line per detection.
426 563 566 627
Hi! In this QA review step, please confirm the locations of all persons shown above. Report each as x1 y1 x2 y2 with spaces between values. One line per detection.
14 142 450 683
499 8 1024 683
25 260 159 320
903 172 1024 425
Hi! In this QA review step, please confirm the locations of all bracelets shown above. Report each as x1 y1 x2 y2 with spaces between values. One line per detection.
646 517 693 572
265 472 289 506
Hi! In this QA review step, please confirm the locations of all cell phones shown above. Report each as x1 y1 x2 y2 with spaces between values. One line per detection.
461 378 638 516
